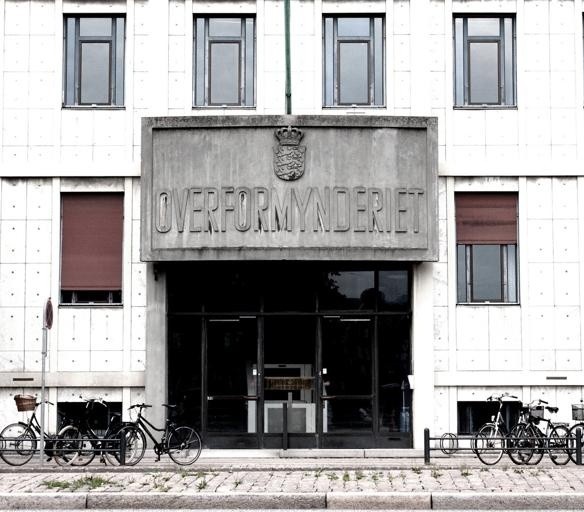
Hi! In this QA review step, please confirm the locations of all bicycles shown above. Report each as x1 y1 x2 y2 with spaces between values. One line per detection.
474 393 583 465
0 394 201 466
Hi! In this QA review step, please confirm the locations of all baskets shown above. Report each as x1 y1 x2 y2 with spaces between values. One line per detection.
14 393 37 411
572 403 584 422
